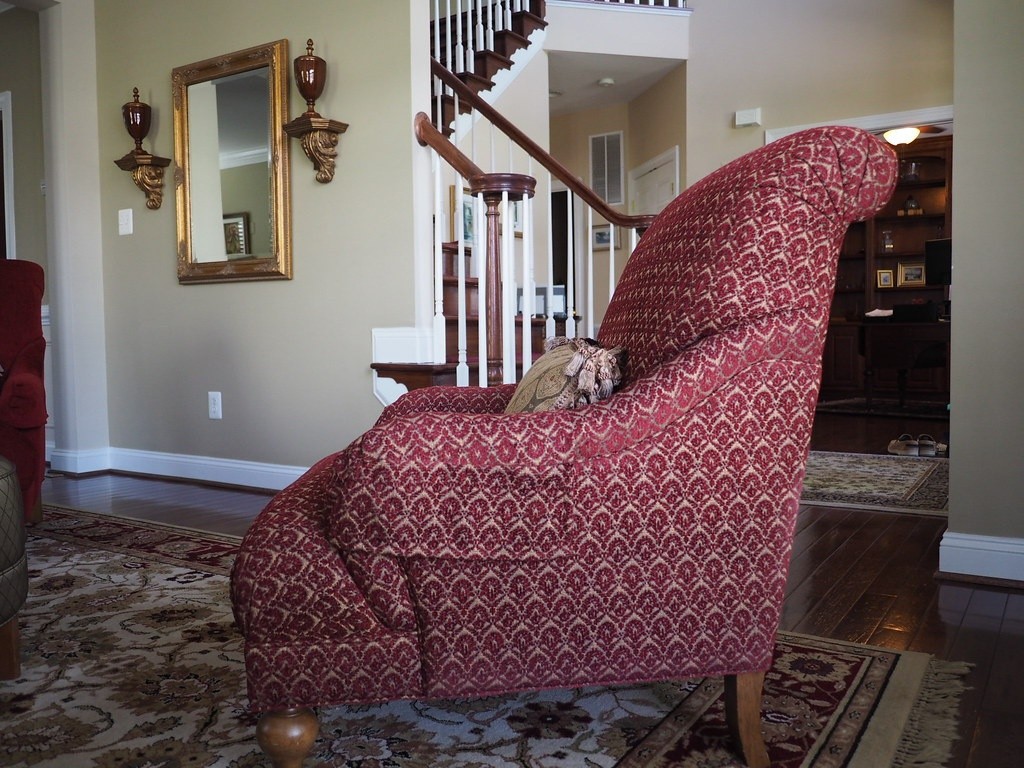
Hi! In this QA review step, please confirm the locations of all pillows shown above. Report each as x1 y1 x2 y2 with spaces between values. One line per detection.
505 336 623 416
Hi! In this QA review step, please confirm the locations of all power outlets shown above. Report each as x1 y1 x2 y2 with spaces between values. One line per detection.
208 391 222 420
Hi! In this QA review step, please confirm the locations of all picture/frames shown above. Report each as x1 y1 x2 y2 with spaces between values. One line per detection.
448 184 477 244
877 269 894 287
898 261 926 288
223 211 250 255
592 224 622 251
497 200 523 238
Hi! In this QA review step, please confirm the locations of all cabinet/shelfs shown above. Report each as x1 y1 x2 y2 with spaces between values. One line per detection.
868 134 953 415
814 218 869 415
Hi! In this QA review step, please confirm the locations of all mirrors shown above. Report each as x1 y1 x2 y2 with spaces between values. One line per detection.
170 38 292 284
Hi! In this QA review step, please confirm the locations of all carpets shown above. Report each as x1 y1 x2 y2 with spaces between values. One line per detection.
0 499 975 768
801 450 949 515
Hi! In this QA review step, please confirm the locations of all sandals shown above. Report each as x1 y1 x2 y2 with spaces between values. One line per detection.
888 434 919 456
917 434 936 456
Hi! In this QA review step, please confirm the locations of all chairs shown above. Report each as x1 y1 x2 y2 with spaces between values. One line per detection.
0 258 47 520
228 125 899 768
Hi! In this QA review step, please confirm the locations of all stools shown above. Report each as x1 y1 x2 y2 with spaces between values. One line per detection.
0 460 29 626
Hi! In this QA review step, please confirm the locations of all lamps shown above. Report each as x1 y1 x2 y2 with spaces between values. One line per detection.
884 127 924 152
114 87 171 210
281 36 349 183
